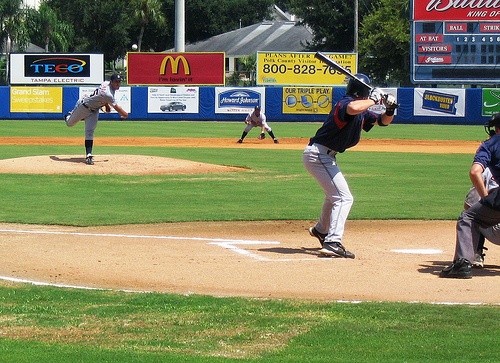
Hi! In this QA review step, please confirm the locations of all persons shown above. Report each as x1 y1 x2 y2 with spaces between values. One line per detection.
236 105 278 143
303 74 395 259
441 111 500 279
65 73 128 165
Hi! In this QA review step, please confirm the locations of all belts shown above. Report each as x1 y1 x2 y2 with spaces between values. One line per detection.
81 100 96 112
308 142 336 159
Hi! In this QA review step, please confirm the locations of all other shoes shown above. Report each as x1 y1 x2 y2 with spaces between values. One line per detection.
438 258 472 279
472 253 486 267
238 139 243 143
86 154 94 164
273 138 278 143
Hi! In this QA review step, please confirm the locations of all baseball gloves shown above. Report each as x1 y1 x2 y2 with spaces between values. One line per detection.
259 133 265 140
100 103 111 113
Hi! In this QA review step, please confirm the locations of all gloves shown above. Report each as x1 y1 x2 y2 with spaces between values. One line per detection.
370 88 386 104
383 93 397 117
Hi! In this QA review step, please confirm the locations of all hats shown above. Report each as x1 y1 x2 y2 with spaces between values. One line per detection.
110 73 123 81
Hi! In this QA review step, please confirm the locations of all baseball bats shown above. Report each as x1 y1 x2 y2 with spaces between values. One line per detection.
313 51 401 109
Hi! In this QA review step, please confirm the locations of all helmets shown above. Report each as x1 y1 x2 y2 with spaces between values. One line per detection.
255 105 260 110
484 113 500 137
344 74 371 100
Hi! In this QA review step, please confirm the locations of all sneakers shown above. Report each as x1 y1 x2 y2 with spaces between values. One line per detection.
309 226 327 247
318 240 356 259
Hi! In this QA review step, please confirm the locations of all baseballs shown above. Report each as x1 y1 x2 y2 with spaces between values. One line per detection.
132 44 137 50
